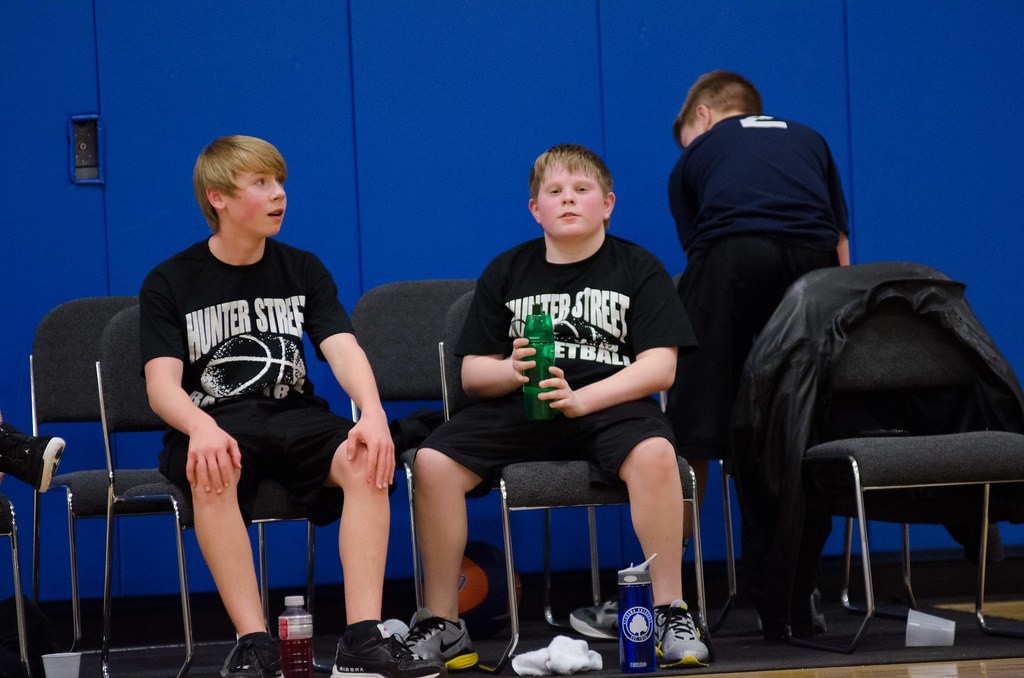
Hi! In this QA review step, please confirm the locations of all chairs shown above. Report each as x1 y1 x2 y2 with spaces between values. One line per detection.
92 296 346 678
434 282 715 674
344 276 607 632
0 492 36 678
720 260 1024 654
24 293 320 652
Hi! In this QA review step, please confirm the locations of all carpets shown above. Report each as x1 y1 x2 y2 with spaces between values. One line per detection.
71 602 1024 678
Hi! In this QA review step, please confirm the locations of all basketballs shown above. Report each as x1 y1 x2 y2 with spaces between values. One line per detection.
420 541 523 637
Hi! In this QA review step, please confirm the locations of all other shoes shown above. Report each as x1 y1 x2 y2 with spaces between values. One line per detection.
759 589 825 637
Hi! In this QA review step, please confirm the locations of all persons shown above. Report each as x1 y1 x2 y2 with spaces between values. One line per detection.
139 136 448 678
407 143 714 667
665 71 852 642
0 410 67 493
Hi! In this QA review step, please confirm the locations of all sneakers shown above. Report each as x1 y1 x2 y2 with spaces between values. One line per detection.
331 619 446 678
221 634 282 678
0 421 64 494
652 600 709 662
401 608 478 672
570 600 621 640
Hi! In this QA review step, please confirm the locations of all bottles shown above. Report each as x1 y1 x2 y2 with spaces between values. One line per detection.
523 314 556 420
617 552 659 673
279 596 313 678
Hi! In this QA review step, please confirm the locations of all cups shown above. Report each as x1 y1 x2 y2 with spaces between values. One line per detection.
384 618 410 638
905 608 956 647
41 653 82 678
